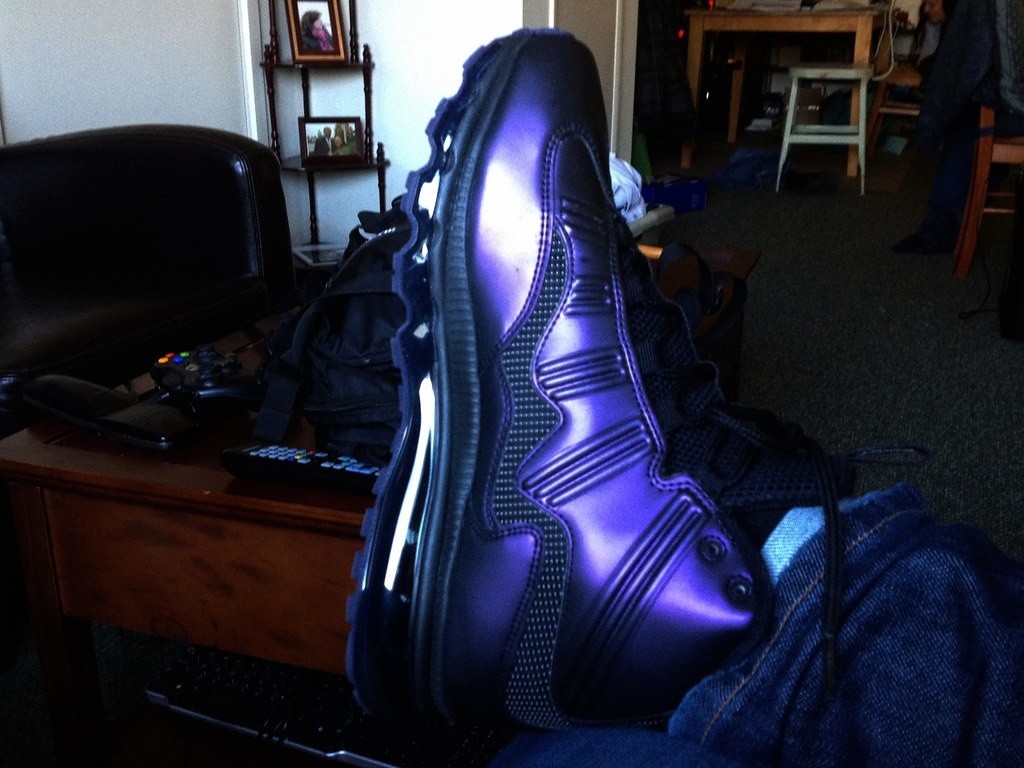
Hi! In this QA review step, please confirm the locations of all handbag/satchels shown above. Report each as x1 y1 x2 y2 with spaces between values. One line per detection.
251 199 409 500
649 227 762 393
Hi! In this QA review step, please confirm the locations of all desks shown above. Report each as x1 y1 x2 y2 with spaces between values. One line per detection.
682 10 880 178
0 303 382 768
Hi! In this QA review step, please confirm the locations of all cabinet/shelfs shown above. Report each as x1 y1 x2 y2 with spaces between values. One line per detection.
259 0 389 265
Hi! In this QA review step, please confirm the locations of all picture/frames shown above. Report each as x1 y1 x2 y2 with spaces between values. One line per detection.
284 0 348 64
293 244 348 267
298 118 364 167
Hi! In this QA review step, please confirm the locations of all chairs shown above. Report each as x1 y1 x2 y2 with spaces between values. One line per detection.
637 238 762 393
864 28 923 157
952 106 1023 280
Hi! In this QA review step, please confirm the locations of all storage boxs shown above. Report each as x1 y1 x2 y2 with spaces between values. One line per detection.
640 176 709 214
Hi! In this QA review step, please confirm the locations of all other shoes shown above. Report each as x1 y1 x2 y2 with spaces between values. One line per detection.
891 198 960 255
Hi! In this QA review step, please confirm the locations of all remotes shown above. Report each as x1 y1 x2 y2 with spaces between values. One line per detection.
221 441 380 496
149 351 249 427
20 372 190 454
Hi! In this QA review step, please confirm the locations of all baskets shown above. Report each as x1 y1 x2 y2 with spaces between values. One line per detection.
784 87 821 124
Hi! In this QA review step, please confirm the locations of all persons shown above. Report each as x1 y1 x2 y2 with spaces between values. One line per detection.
300 10 332 50
314 128 350 156
890 0 1024 257
345 26 1024 767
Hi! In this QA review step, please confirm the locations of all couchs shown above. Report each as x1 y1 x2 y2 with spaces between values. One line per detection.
0 127 296 430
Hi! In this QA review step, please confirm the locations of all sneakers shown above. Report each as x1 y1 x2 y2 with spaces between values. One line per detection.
340 26 869 739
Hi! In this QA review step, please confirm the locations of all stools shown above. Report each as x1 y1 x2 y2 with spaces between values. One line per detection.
774 63 874 196
